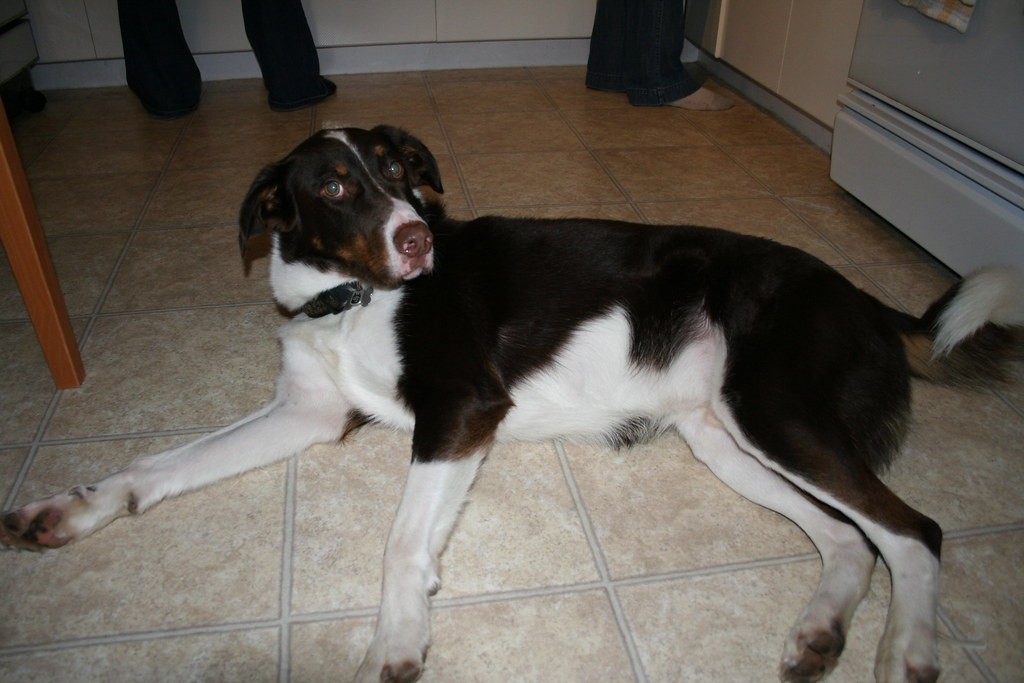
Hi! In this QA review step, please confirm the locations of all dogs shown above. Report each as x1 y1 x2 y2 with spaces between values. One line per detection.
0 124 1024 683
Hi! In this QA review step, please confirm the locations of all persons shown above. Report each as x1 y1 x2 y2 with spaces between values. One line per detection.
585 0 738 110
116 0 338 120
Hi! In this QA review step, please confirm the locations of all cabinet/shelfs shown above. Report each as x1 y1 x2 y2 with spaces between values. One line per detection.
1 0 863 149
